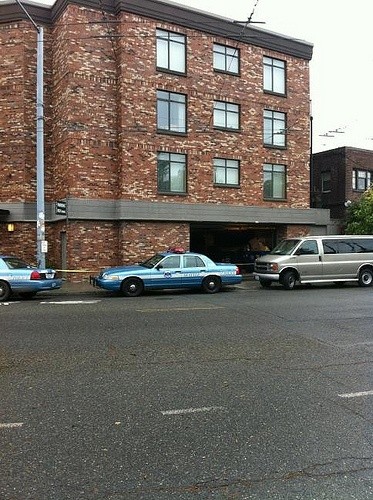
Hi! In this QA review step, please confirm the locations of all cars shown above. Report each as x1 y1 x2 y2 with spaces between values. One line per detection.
91 247 243 296
0 256 62 301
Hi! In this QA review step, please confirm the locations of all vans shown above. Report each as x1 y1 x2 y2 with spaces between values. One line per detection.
252 235 373 289
